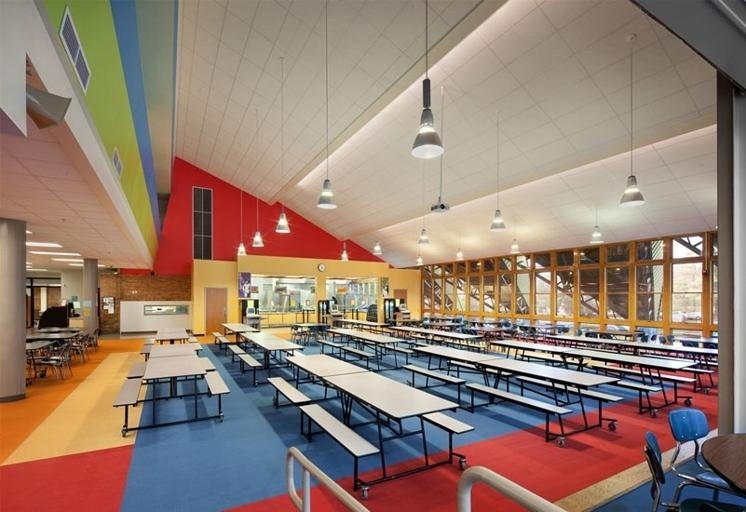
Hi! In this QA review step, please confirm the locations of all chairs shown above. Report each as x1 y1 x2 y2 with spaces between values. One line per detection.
644 431 746 512
665 406 745 500
25 327 100 385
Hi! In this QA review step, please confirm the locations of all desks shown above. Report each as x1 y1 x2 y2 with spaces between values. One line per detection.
702 432 746 494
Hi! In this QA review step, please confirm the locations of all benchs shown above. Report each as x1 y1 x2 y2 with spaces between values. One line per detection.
265 315 719 501
212 322 304 386
112 314 231 438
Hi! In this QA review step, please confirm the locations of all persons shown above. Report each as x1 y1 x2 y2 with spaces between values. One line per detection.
243 279 250 297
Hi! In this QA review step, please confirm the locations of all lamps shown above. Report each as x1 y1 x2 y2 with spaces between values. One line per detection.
316 1 338 210
490 120 505 233
618 33 645 207
237 1 291 258
340 202 605 266
411 2 451 246
